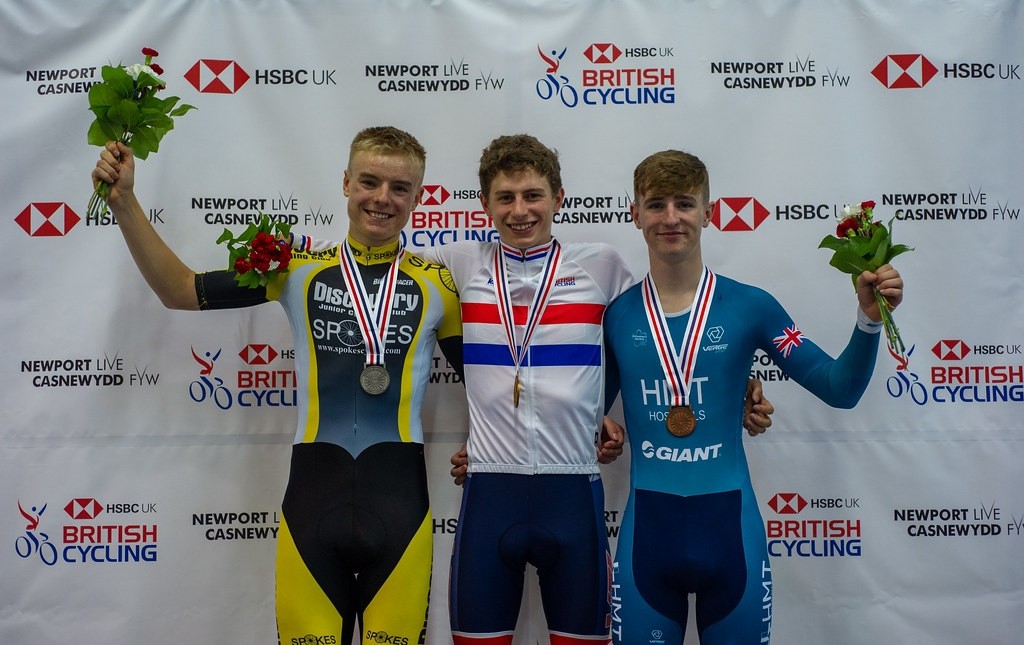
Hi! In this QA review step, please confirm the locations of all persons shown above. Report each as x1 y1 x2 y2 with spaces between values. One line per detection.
275 132 774 645
90 127 624 645
450 149 904 645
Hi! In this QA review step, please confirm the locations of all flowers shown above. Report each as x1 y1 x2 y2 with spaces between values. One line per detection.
214 206 295 291
81 46 199 230
817 200 918 372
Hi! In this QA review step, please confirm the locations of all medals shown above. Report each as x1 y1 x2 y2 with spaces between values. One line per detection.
667 407 696 436
513 376 520 408
360 365 390 395
